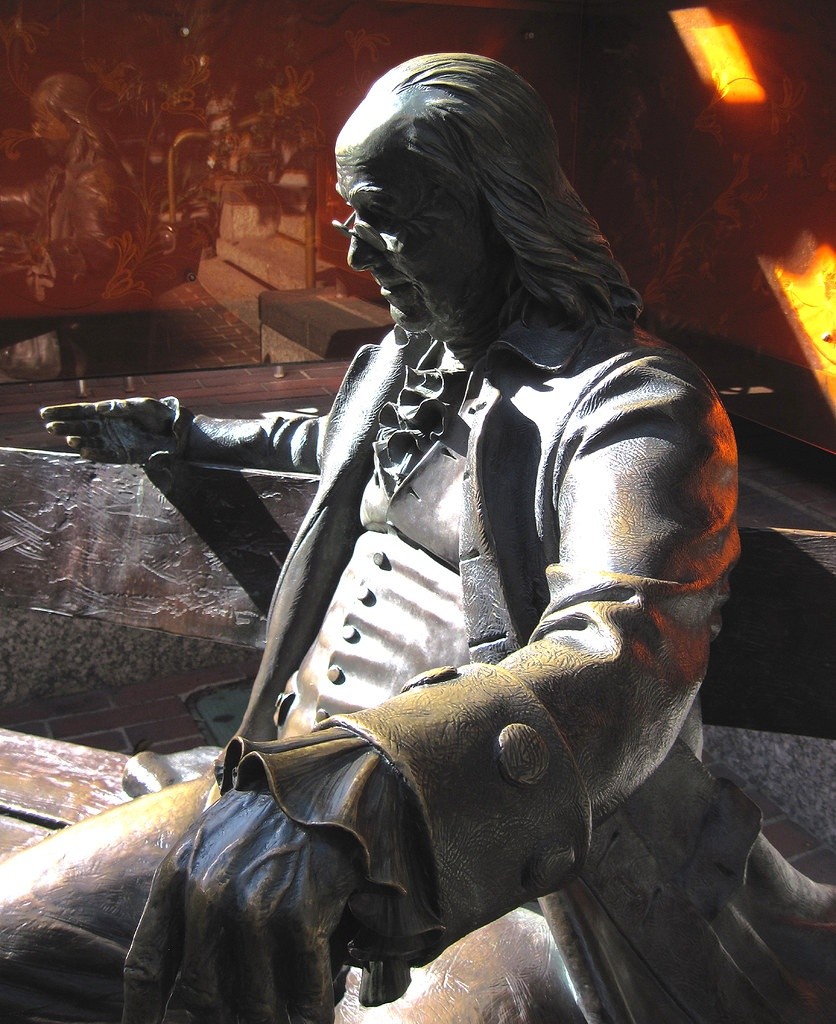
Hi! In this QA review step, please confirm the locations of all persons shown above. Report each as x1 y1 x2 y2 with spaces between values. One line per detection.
0 73 149 303
0 53 834 1024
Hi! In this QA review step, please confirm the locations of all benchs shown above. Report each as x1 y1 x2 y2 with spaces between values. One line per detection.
254 286 394 364
0 441 836 861
